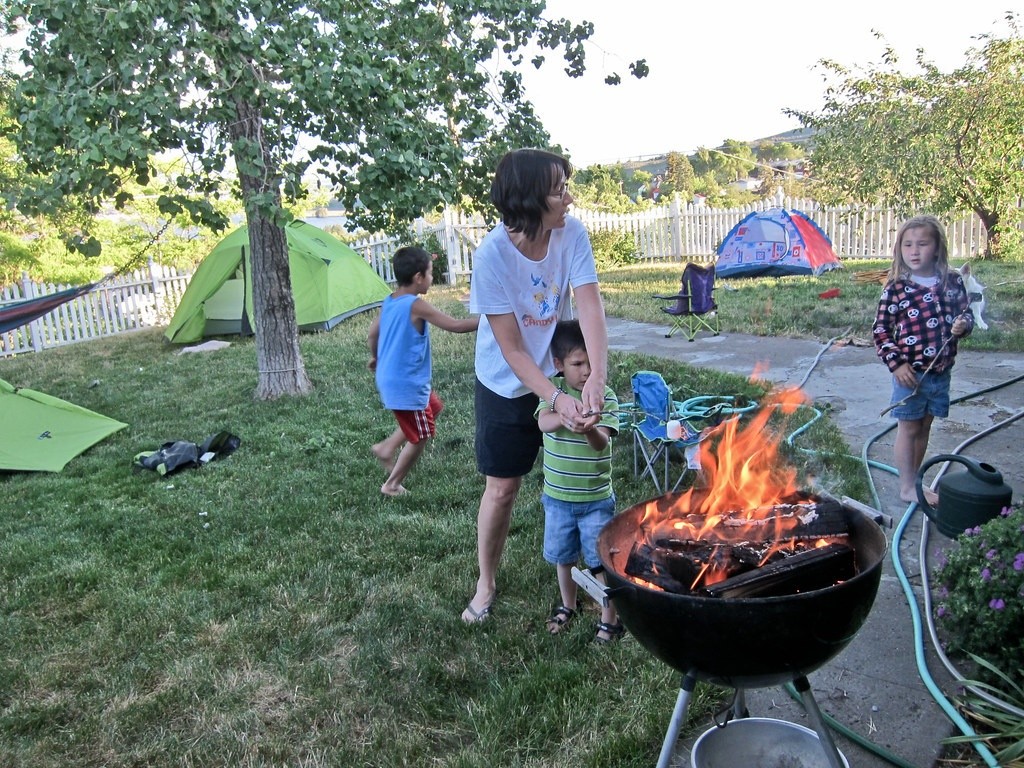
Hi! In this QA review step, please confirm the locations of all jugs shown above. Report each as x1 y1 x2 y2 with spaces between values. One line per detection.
915 453 1014 541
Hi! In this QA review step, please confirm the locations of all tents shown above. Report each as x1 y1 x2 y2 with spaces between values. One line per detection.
164 216 395 344
0 375 131 473
715 208 842 276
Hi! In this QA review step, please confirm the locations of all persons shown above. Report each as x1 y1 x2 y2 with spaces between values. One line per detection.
872 215 975 508
533 319 624 646
459 148 607 625
367 246 479 498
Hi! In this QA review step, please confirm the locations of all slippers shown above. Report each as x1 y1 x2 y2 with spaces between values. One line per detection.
461 600 489 627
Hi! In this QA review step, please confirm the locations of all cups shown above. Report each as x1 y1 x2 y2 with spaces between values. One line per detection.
667 419 681 440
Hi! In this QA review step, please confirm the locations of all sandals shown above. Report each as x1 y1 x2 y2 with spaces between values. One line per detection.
590 618 627 647
544 600 583 635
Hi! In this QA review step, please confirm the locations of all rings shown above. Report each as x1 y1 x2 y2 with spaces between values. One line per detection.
569 423 573 427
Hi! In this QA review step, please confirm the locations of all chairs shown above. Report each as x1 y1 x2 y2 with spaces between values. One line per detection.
651 262 720 342
630 370 702 496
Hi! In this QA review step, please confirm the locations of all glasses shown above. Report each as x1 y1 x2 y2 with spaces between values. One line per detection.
548 183 569 198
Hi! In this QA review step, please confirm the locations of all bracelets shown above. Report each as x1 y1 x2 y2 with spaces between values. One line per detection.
550 389 564 412
